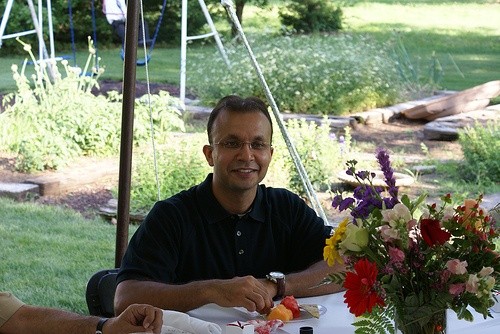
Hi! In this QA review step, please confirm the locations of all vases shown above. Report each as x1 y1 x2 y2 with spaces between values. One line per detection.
393 306 447 334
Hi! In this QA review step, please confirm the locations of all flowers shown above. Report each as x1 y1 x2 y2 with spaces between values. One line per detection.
310 147 500 334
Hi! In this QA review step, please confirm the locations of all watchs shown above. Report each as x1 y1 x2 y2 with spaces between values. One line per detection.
266 271 287 301
95 317 109 333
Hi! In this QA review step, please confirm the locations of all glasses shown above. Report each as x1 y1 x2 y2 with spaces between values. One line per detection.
211 138 271 150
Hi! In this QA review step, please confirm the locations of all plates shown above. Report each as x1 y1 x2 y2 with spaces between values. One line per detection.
261 304 328 323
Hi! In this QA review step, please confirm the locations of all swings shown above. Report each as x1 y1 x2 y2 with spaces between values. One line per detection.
120 0 167 66
68 0 98 77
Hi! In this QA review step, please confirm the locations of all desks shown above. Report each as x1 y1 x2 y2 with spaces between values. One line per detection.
184 290 500 334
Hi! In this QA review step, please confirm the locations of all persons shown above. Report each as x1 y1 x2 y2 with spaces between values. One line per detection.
112 94 352 317
104 0 126 46
0 288 164 334
